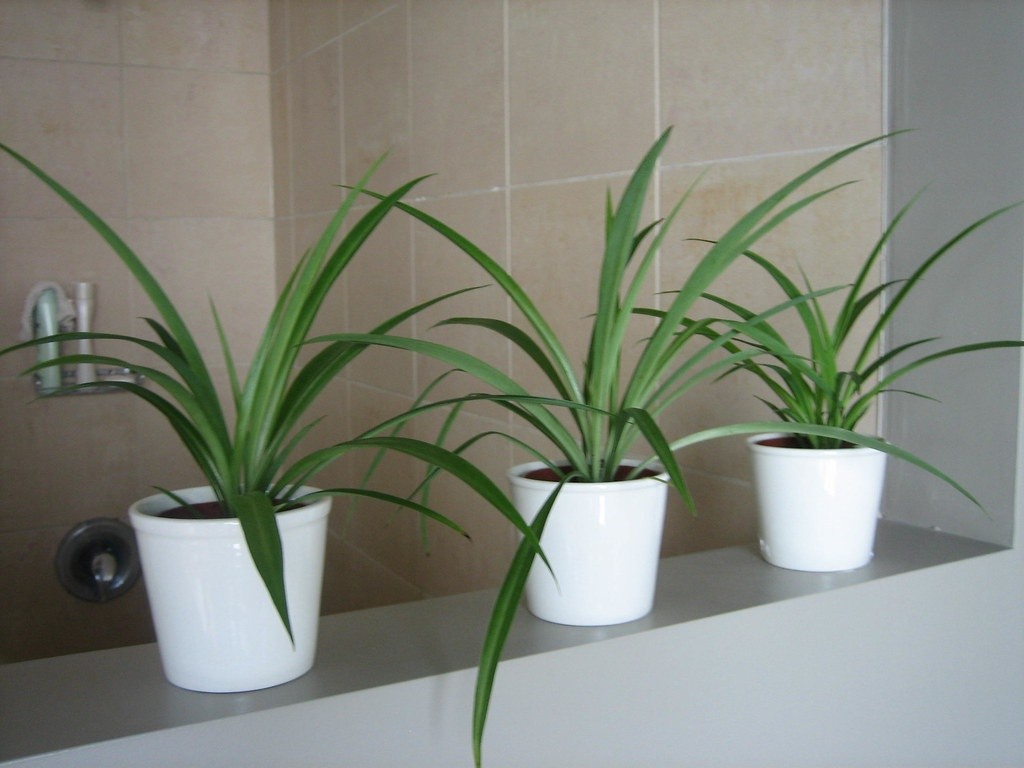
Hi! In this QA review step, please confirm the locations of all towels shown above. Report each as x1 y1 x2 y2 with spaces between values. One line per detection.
21 280 76 340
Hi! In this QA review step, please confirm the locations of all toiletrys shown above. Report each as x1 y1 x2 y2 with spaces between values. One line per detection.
69 281 97 391
34 293 59 392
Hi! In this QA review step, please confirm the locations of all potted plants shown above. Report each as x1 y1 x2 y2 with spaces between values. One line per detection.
0 140 471 693
588 189 1024 574
314 115 982 768
